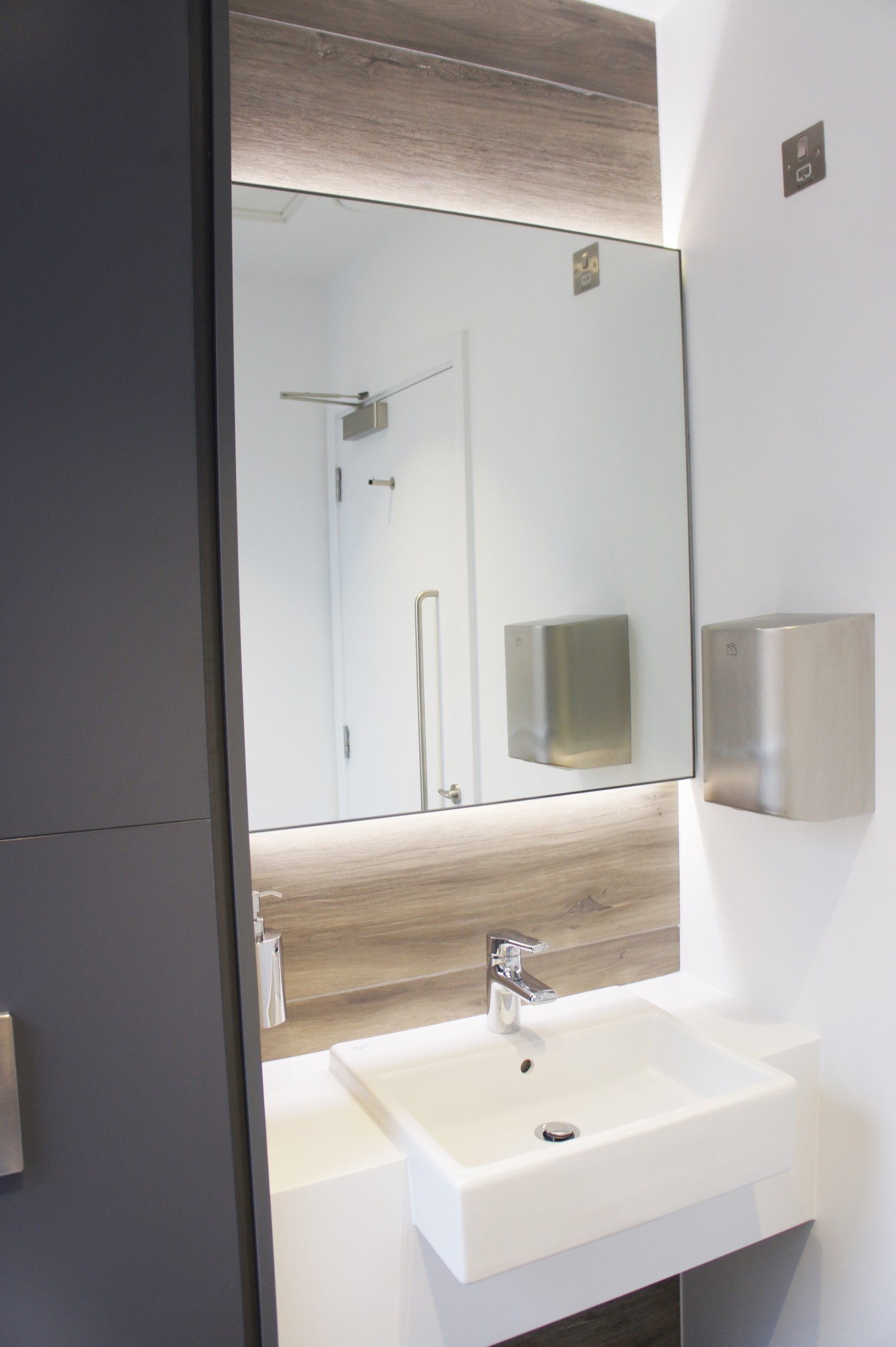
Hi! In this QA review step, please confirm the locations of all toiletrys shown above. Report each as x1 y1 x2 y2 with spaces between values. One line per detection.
251 888 290 1029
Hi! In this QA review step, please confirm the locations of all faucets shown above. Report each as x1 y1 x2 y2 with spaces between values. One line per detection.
484 928 560 1037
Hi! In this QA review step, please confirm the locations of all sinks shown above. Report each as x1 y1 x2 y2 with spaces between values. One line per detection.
329 984 803 1285
